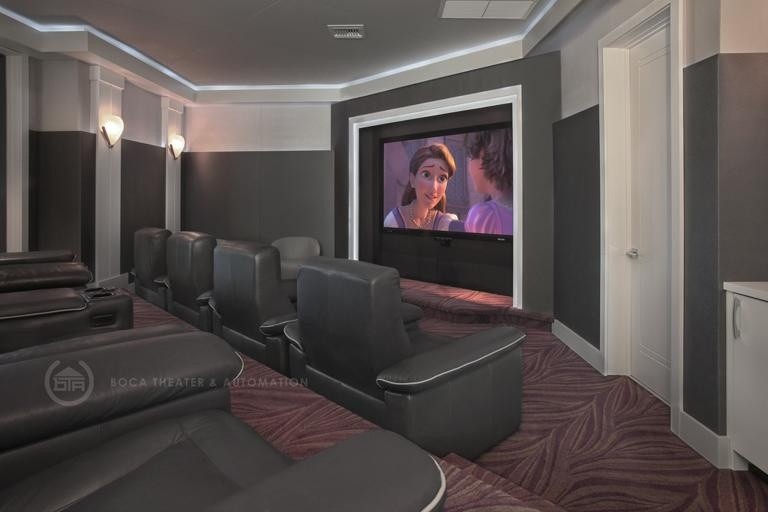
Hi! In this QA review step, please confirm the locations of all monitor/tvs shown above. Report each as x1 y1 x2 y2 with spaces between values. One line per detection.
377 119 513 242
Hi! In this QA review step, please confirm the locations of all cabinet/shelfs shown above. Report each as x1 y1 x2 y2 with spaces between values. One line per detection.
721 283 768 475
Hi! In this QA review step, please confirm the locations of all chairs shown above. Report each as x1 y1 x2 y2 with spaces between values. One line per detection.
0 225 527 512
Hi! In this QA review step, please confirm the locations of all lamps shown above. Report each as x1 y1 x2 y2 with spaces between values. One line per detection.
101 115 124 148
170 135 185 160
